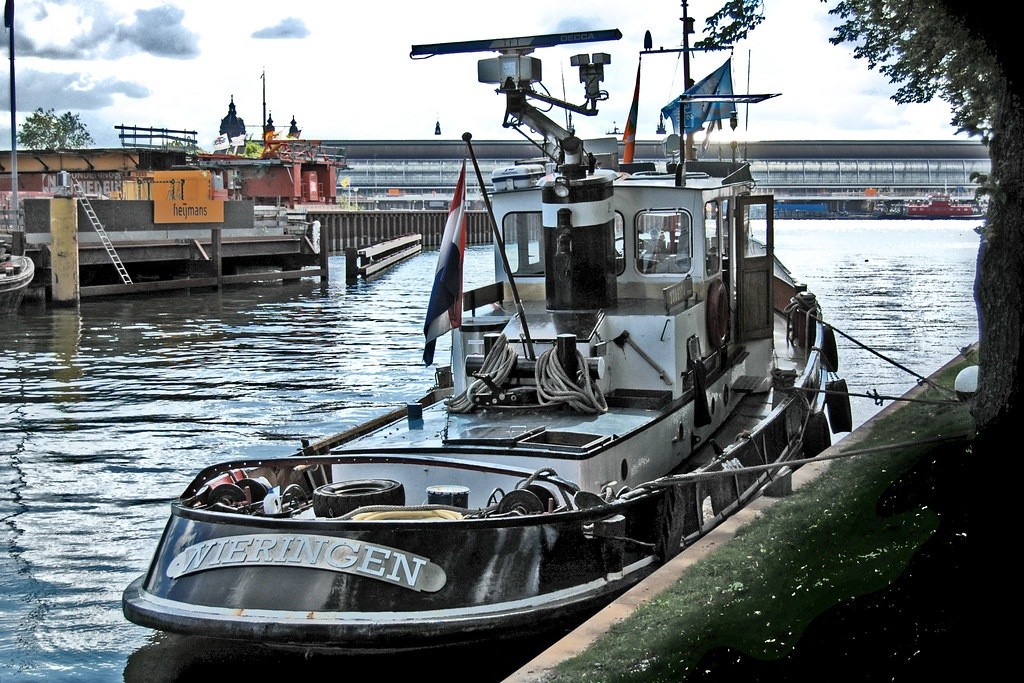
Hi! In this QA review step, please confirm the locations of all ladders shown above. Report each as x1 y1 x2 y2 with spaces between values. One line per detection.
70 176 134 285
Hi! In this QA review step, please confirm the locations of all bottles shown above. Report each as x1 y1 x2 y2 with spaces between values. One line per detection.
263 488 281 514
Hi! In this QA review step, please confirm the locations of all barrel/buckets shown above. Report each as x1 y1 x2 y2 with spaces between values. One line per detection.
436 365 451 388
426 484 470 510
6 267 14 277
14 265 22 275
407 403 422 419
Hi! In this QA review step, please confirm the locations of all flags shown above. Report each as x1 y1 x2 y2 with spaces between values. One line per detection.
663 57 735 133
422 157 467 368
340 177 349 189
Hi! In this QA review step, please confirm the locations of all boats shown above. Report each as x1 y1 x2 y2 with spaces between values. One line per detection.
120 0 853 656
0 1 356 301
900 192 975 219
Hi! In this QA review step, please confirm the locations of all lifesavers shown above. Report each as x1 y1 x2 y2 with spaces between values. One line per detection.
825 378 854 433
800 412 832 458
311 477 406 517
822 323 839 374
